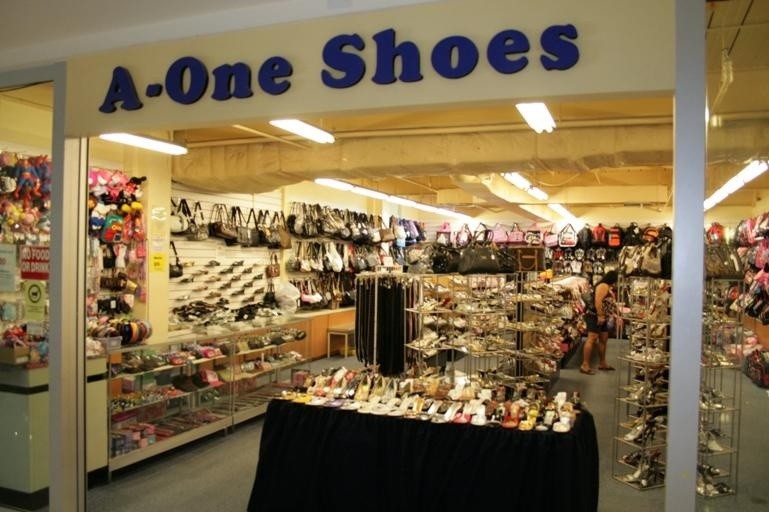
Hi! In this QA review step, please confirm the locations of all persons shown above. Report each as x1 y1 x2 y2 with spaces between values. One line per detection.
579 270 626 375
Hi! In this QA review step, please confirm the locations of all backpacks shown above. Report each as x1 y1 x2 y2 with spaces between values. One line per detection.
734 212 768 326
577 223 672 249
707 225 724 243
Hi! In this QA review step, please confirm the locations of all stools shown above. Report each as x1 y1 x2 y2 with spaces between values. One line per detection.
326 320 354 359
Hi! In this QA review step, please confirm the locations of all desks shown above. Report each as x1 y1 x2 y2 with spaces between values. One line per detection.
258 384 594 512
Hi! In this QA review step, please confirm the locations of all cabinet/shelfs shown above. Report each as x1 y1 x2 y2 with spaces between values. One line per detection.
108 322 309 482
612 266 741 501
404 263 593 380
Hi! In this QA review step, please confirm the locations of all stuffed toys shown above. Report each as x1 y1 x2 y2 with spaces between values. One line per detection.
0 152 147 346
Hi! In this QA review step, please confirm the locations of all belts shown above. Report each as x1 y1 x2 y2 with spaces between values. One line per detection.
354 274 415 377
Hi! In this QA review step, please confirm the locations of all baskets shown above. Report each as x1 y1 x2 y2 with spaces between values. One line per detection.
119 326 310 373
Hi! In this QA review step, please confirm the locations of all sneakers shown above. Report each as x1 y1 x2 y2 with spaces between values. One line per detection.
177 260 264 304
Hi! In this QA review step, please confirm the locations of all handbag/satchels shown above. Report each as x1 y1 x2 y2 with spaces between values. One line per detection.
168 264 184 278
268 264 280 278
407 239 546 275
744 351 769 389
435 227 578 248
264 292 277 304
617 244 744 280
605 315 616 332
287 215 427 310
170 212 292 249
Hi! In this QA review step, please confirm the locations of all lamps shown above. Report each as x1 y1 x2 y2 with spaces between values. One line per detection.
104 132 190 157
269 117 337 144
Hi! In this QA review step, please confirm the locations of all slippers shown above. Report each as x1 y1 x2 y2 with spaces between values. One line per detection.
581 364 615 374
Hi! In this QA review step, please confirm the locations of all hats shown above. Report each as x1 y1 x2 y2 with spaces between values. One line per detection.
171 368 225 392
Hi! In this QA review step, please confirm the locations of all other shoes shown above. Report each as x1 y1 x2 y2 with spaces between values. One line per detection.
279 366 582 434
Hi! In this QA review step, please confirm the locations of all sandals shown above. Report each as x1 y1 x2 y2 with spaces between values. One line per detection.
412 280 550 394
620 279 734 497
